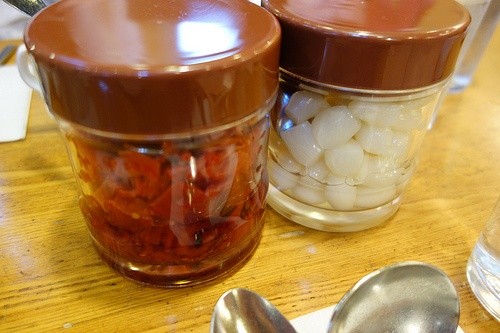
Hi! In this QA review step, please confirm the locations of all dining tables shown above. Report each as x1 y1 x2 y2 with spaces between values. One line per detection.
0 39 500 333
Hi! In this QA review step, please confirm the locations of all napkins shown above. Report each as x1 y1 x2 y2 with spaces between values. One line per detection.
0 65 32 143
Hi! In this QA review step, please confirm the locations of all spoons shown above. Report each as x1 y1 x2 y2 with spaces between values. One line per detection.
210 288 298 333
326 262 461 333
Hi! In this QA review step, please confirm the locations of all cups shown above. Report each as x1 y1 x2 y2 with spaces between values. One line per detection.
465 199 500 321
